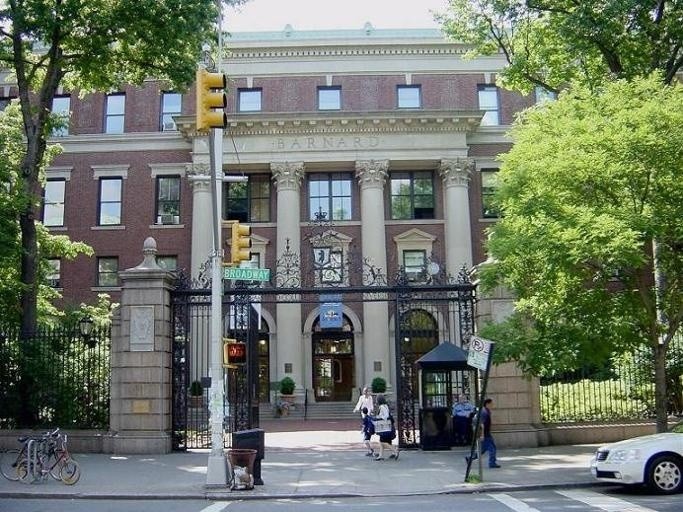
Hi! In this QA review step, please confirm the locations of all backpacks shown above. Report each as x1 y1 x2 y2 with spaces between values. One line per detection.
364 417 374 435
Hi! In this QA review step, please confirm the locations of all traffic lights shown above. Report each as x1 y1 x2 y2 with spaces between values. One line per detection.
230 220 253 266
193 62 230 135
223 341 247 368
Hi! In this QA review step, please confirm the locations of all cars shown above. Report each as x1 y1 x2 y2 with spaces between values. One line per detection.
590 416 682 495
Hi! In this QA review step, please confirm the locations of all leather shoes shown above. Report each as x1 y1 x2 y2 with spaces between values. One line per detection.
465 457 471 466
489 464 500 468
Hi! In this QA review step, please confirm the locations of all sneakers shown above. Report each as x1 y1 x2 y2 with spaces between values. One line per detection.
366 449 398 461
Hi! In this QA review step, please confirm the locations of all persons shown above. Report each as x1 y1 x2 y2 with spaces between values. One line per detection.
359 406 376 457
386 413 396 459
371 392 399 462
450 392 477 447
351 386 374 418
464 397 501 468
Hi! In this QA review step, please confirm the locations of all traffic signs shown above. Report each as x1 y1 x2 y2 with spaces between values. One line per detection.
224 267 271 281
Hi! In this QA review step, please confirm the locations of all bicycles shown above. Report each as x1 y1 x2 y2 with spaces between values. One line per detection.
0 427 82 489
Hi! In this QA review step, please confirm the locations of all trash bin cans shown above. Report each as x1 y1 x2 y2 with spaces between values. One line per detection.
227 449 258 490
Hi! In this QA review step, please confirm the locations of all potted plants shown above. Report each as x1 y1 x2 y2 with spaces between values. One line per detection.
186 381 203 407
278 377 295 404
369 377 387 403
